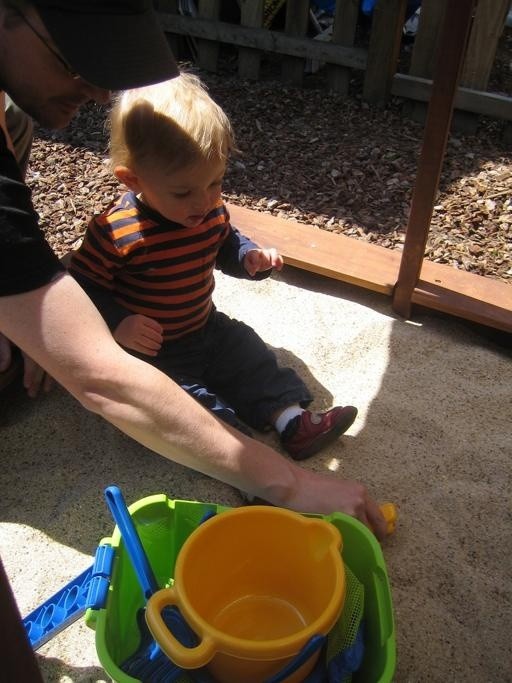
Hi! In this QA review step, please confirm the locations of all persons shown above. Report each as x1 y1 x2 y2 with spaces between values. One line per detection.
1 1 388 541
67 73 358 503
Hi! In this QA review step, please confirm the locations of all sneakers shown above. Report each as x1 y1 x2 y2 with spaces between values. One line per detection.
281 406 357 461
